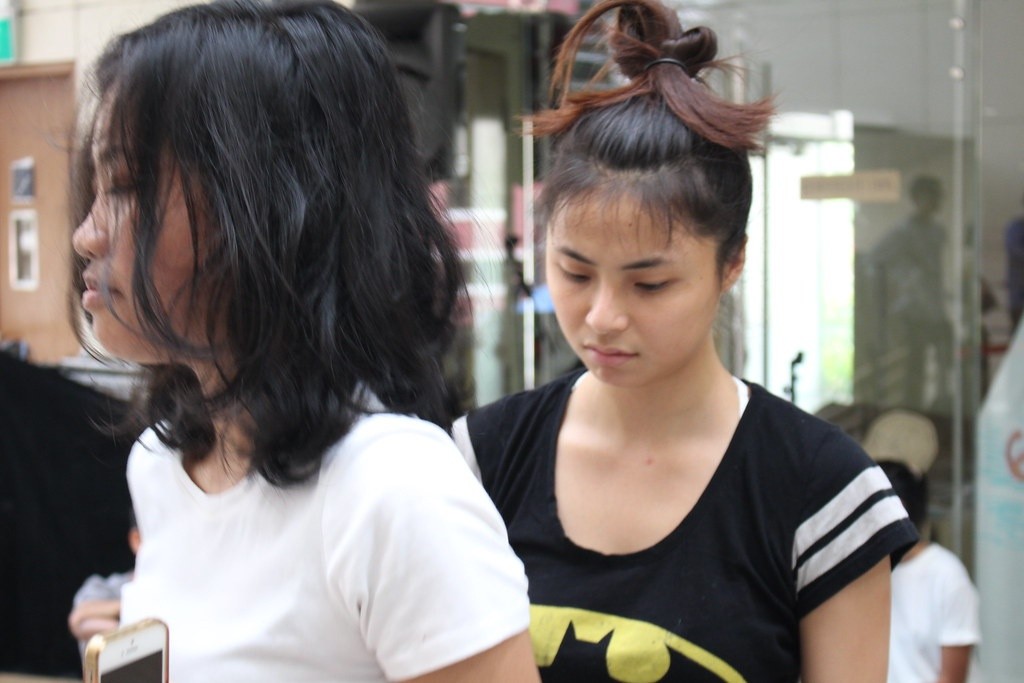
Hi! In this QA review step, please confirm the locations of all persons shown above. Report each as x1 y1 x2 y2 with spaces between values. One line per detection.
0 0 982 683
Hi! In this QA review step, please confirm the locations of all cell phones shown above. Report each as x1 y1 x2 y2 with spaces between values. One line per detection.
80 618 169 683
